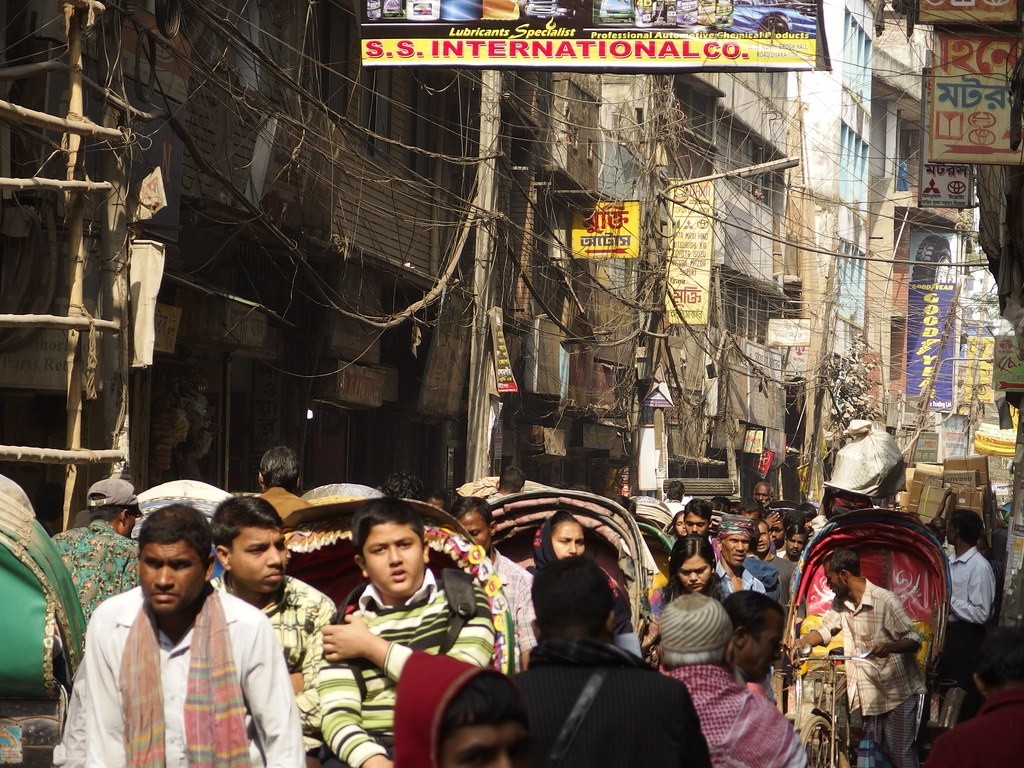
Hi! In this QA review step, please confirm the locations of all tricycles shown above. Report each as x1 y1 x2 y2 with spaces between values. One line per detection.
0 471 678 767
770 507 952 768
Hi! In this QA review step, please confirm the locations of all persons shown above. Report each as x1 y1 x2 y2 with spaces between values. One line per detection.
49 478 141 625
316 470 538 768
391 652 534 768
656 593 808 768
510 557 713 768
53 504 306 768
887 501 896 511
923 627 1024 768
525 510 633 647
612 481 824 705
924 487 1005 696
486 466 525 505
790 549 922 768
208 496 337 768
255 446 313 528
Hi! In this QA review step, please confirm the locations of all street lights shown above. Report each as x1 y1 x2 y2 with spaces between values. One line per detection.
624 156 802 495
907 358 994 466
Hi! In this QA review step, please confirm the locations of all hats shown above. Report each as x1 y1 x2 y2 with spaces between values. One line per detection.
660 592 732 652
86 478 144 517
